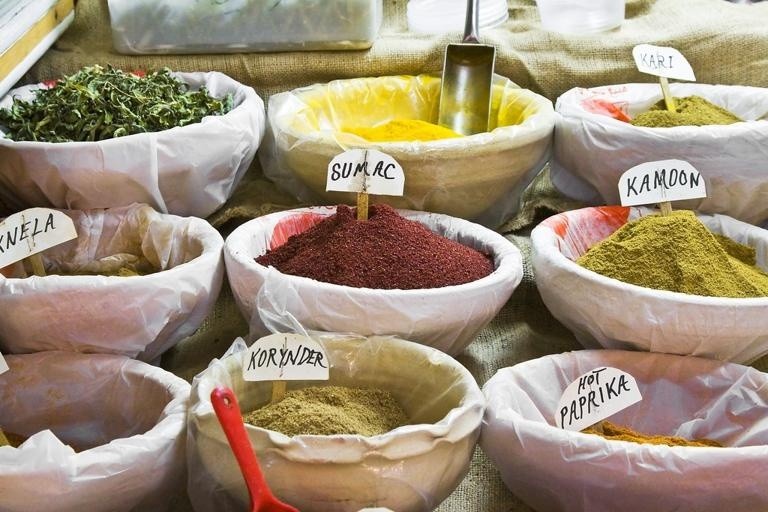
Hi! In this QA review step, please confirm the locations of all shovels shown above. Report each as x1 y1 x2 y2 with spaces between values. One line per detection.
211 386 298 512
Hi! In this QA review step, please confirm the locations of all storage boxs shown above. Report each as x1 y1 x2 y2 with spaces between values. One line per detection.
108 0 385 57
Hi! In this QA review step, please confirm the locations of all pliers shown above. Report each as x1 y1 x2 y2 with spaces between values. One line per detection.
438 0 496 133
